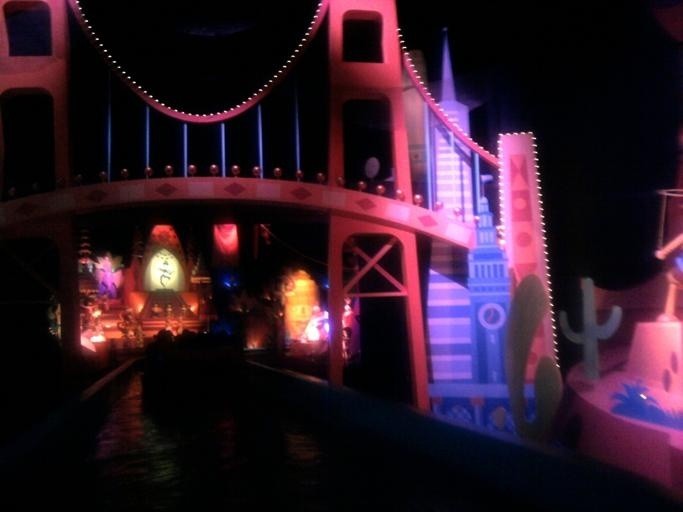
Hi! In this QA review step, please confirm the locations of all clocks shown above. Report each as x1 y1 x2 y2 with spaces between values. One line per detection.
475 302 507 333
